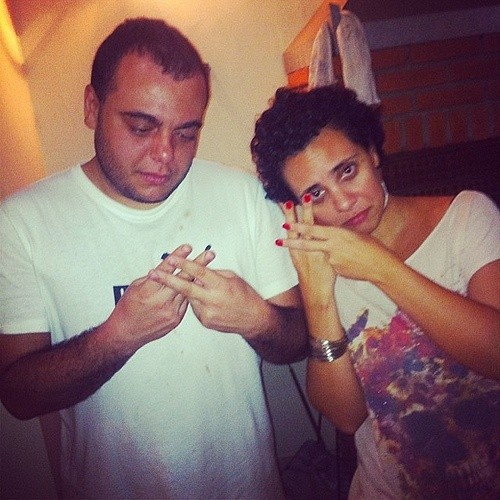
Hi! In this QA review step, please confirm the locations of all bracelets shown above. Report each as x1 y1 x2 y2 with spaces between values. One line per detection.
306 334 350 363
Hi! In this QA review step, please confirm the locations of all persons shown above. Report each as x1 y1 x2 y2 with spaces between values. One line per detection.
0 18 307 500
249 83 500 500
